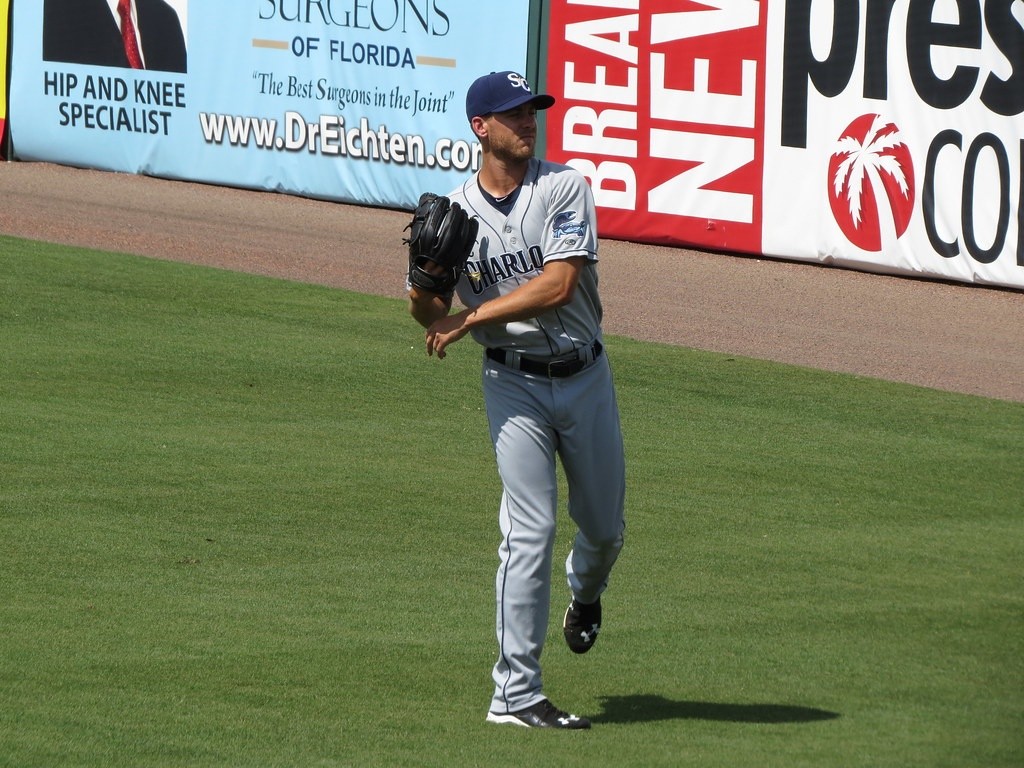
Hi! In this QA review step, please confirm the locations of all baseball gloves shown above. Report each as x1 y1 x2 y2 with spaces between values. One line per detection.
408 191 480 295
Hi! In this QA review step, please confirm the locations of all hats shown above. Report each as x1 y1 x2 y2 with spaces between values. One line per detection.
466 70 555 122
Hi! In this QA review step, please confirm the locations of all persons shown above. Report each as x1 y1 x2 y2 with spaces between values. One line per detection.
401 68 624 731
44 0 188 73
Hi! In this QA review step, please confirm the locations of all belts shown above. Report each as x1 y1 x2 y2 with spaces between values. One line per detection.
486 339 602 379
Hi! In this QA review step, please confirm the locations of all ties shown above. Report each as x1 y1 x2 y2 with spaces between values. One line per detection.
117 0 144 69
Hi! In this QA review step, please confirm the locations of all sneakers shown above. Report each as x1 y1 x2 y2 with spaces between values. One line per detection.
563 590 602 654
485 699 591 731
